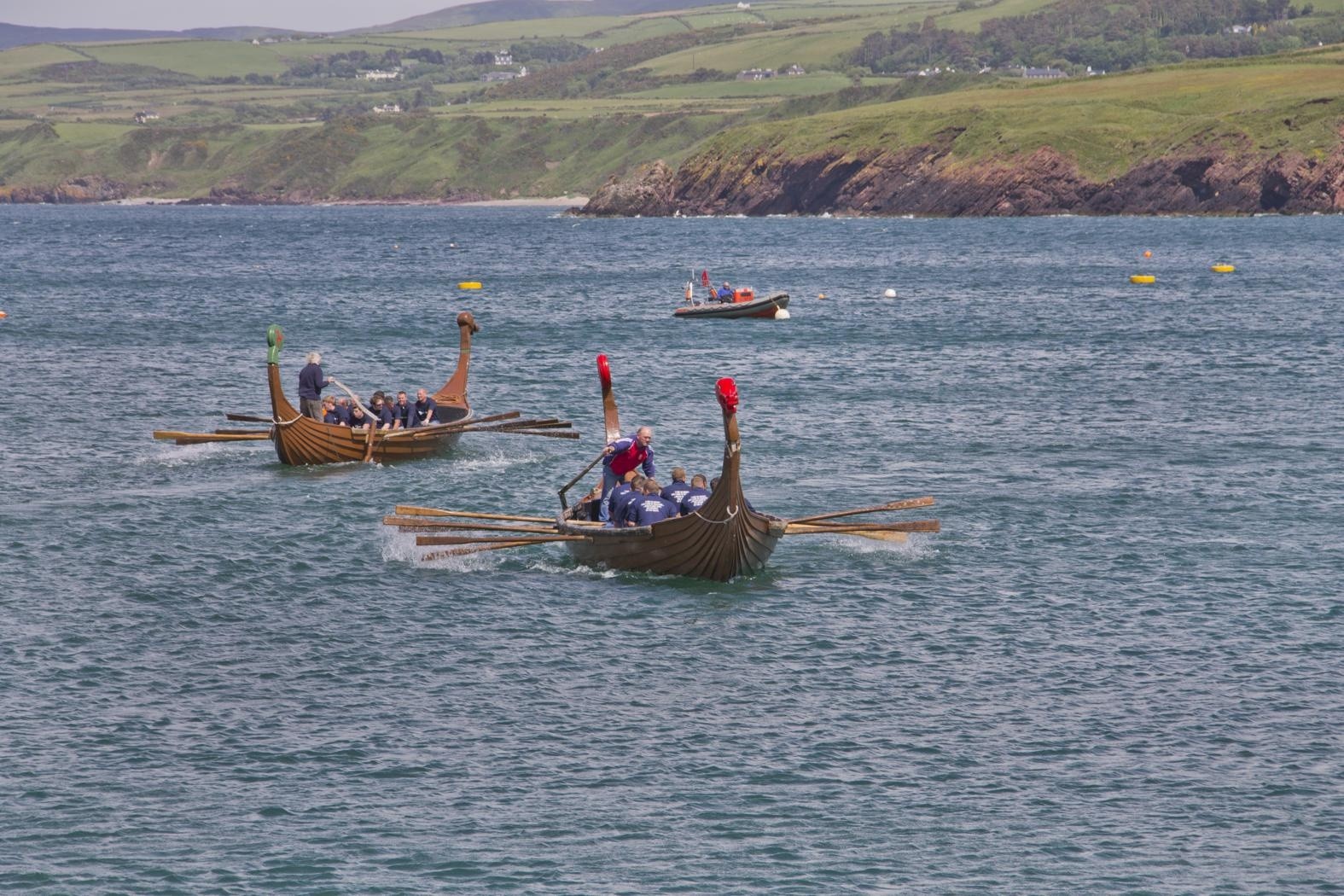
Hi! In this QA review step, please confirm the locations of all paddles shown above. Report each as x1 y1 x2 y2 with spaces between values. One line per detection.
155 414 273 444
383 411 581 439
385 505 593 560
785 496 941 543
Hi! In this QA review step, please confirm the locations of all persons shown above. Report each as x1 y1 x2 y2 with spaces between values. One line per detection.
322 388 439 432
717 281 734 303
599 427 756 528
299 352 336 422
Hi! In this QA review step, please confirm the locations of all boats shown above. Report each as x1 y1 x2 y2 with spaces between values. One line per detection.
264 313 482 468
555 354 789 584
671 279 791 320
